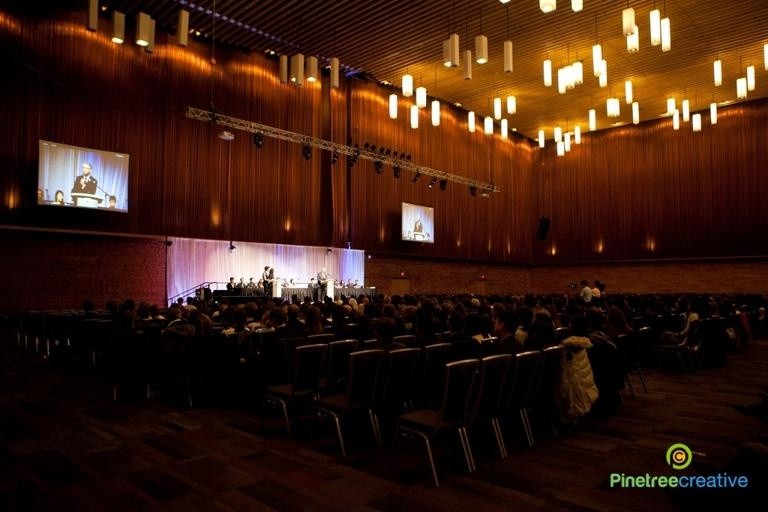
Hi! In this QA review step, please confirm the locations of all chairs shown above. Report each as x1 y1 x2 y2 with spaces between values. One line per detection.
0 274 766 491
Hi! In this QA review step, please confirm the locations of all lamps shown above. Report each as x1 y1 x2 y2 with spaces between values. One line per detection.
78 1 767 201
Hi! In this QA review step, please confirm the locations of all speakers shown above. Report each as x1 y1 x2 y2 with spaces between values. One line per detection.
536 217 551 240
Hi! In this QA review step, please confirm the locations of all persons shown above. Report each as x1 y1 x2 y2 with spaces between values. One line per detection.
37 189 46 205
108 195 116 209
81 264 768 364
403 214 431 242
70 162 97 207
51 190 67 205
